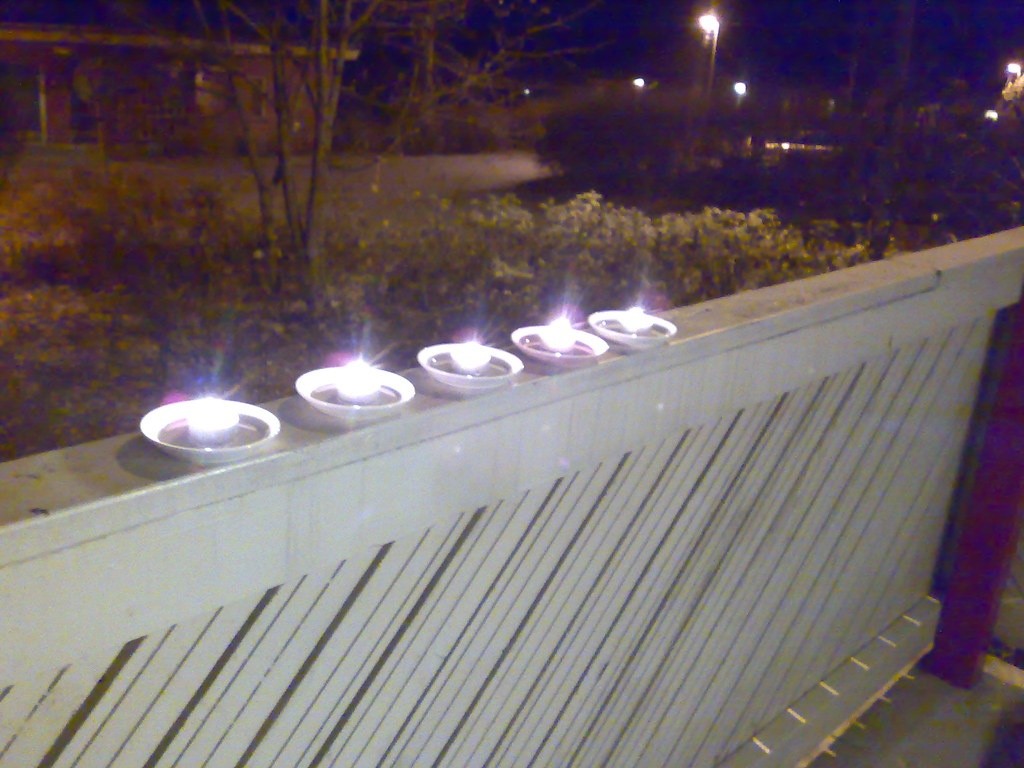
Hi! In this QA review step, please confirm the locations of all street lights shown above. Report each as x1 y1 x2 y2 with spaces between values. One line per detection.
697 8 722 114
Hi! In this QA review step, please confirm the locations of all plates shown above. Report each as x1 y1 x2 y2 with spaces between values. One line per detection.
296 367 415 421
589 310 677 350
418 344 524 389
512 326 610 365
140 400 280 466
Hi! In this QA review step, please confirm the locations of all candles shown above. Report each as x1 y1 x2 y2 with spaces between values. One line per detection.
620 303 655 333
333 356 382 405
187 395 240 445
537 313 578 354
450 338 491 372
